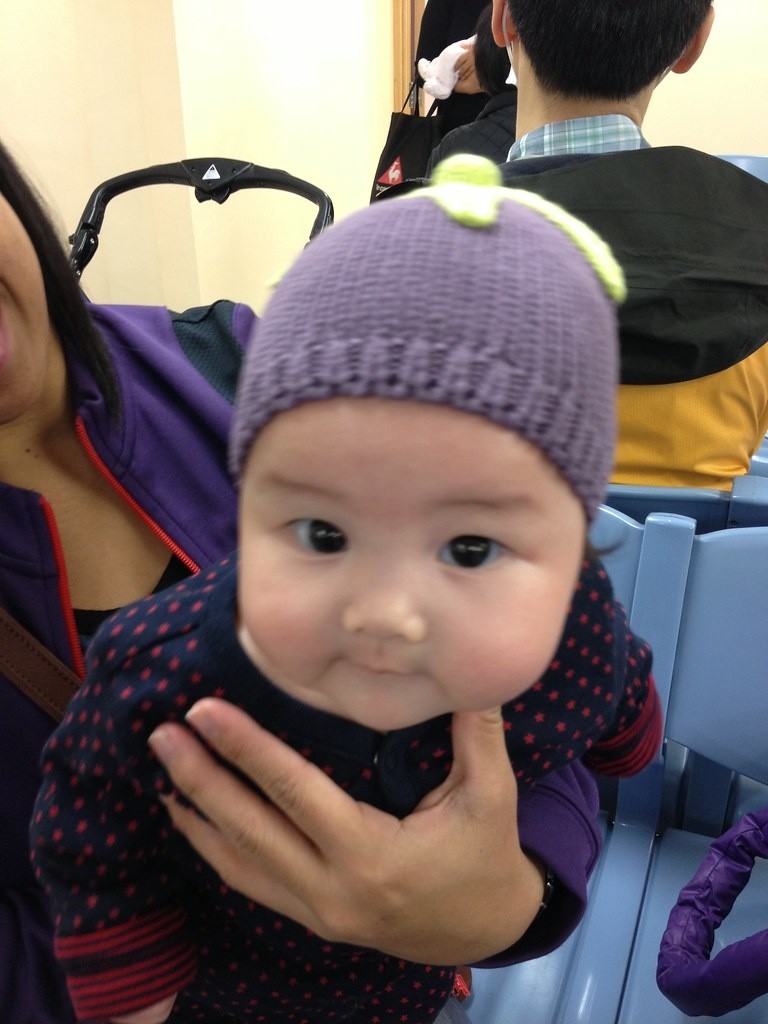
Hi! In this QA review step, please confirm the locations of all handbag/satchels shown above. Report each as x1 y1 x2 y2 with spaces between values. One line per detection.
369 76 440 205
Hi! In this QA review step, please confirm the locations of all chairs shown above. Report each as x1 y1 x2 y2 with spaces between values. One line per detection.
439 476 768 1024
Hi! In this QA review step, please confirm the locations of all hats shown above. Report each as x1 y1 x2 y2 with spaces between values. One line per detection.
229 152 628 523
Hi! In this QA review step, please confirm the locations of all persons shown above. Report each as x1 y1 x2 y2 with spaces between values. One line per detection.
414 0 492 129
385 0 768 488
0 141 602 1022
29 156 662 1024
424 5 517 179
